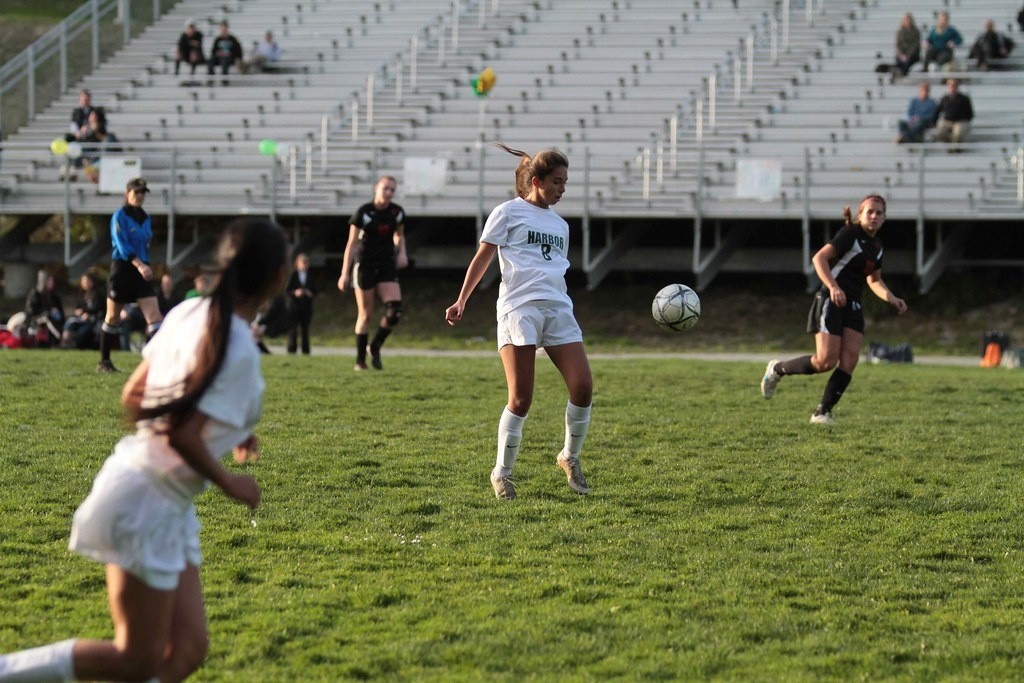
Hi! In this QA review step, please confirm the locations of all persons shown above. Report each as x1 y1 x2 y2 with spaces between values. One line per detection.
97 175 164 375
21 267 273 356
445 140 593 504
759 191 908 429
172 17 282 88
887 9 1016 87
285 250 321 357
898 79 977 152
56 87 123 196
0 214 291 683
337 174 408 373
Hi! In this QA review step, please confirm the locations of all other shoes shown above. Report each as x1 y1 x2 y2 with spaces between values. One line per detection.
370 358 382 370
353 363 368 371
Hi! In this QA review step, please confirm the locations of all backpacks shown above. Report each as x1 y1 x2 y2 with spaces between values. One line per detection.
983 329 1010 361
1000 348 1024 368
979 342 1001 368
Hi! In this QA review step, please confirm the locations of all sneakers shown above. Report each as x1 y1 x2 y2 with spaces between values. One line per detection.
557 448 588 495
490 468 519 501
810 413 835 425
95 361 122 373
761 359 783 399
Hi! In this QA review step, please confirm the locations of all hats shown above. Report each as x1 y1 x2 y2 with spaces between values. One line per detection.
127 177 150 194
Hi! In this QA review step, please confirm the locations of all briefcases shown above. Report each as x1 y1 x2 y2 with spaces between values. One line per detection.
867 341 913 363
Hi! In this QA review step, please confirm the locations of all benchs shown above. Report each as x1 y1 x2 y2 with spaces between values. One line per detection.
0 0 1024 220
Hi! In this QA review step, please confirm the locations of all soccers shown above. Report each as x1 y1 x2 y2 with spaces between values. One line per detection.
652 284 701 334
